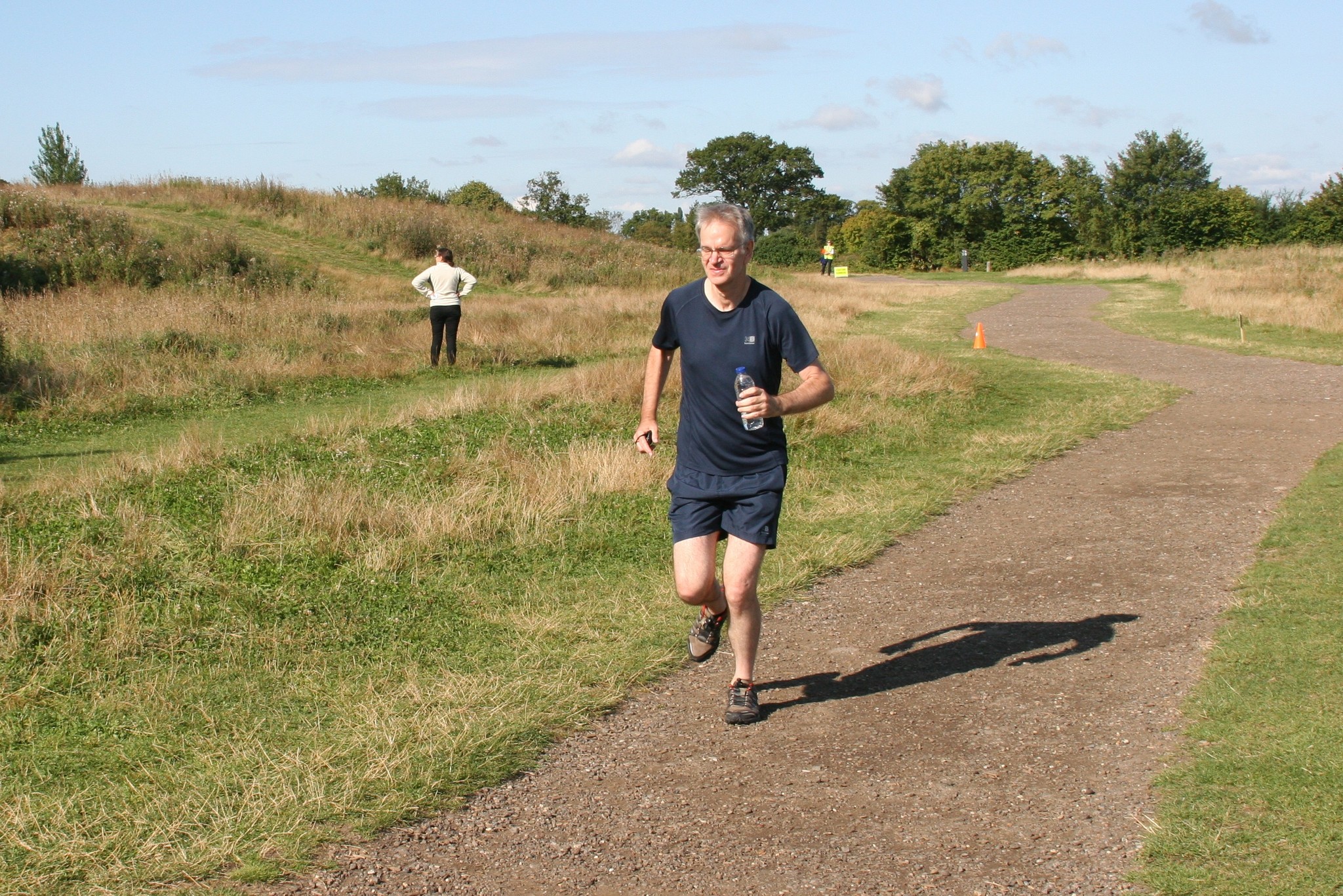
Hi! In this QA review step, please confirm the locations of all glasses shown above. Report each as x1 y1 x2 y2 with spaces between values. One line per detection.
434 254 440 258
697 241 748 260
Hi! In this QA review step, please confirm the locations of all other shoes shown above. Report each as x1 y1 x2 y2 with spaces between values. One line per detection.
821 272 824 275
828 274 830 277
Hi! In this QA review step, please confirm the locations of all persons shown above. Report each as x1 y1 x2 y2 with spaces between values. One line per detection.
819 239 835 277
411 248 477 367
634 201 834 723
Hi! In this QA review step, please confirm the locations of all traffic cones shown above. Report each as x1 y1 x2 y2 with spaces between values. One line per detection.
973 322 988 350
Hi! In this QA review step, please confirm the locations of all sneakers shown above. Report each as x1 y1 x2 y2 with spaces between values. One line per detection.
687 584 730 662
725 676 760 724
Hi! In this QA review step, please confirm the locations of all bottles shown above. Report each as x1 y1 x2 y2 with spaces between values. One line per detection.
734 367 764 431
